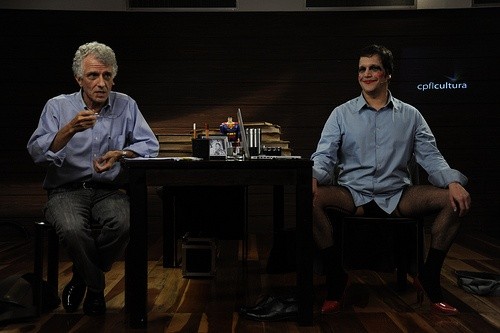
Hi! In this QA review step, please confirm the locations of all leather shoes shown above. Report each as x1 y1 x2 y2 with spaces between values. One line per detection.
62 261 86 314
237 288 299 323
83 286 106 316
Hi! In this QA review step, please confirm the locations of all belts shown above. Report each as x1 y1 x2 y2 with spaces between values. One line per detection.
56 180 118 191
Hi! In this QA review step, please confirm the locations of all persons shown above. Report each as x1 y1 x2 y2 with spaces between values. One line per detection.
309 44 472 317
26 42 160 317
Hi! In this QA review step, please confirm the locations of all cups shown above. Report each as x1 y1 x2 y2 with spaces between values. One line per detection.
192 138 210 160
234 147 245 160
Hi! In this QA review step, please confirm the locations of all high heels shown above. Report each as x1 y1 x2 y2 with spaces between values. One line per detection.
414 275 460 316
320 269 352 316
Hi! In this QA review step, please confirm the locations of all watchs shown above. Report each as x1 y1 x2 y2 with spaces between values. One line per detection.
117 151 127 163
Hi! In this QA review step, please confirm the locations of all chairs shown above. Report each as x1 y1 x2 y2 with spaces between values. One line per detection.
32 165 127 310
336 213 423 310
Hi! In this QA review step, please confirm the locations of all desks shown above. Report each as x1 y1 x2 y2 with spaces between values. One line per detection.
127 158 313 329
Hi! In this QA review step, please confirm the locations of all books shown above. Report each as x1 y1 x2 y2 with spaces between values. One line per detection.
243 122 293 156
